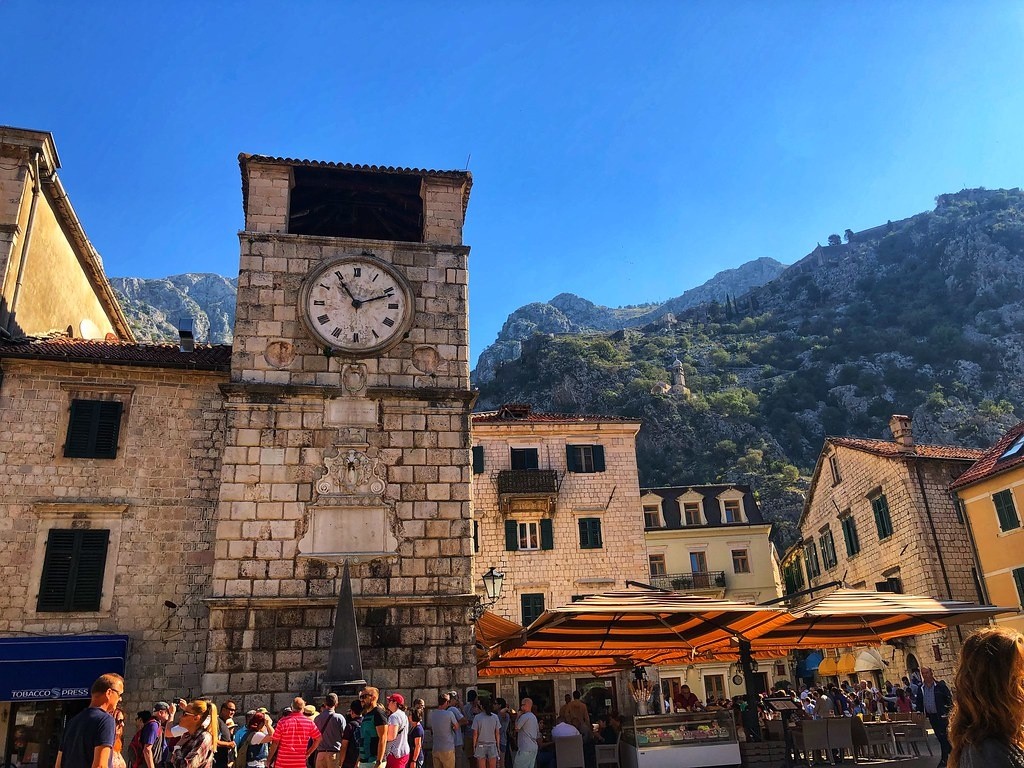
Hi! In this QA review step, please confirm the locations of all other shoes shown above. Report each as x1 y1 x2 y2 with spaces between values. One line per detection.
937 759 947 768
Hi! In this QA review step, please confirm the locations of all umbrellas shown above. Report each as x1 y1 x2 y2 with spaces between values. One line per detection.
744 587 1018 650
478 588 793 672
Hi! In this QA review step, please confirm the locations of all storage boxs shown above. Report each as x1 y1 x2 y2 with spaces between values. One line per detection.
555 734 585 768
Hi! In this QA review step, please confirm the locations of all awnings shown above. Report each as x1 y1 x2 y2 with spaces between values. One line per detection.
0 635 130 702
804 651 822 670
818 657 837 676
475 610 526 659
837 653 855 675
854 649 883 672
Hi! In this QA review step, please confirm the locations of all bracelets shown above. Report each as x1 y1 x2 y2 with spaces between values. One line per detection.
412 759 416 762
376 760 381 766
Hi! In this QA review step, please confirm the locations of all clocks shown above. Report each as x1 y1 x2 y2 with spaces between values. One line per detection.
296 253 416 359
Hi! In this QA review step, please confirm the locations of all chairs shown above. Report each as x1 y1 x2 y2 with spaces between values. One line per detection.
792 718 858 767
849 711 933 761
595 731 621 768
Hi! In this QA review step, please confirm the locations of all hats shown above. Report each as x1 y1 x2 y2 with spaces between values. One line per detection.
245 686 512 729
153 701 170 712
386 694 404 706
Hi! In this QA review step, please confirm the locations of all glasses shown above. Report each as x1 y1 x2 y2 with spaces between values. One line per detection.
225 706 236 713
493 701 498 706
360 694 372 700
135 717 139 721
520 703 527 706
104 686 125 698
182 711 202 721
116 718 125 725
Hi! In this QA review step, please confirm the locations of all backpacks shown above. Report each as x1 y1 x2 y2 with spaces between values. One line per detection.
866 698 878 713
127 719 162 768
235 731 256 768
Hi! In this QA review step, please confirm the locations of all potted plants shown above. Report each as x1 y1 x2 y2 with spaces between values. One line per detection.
671 577 725 590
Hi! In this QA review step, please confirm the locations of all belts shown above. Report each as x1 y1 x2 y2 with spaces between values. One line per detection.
318 749 340 753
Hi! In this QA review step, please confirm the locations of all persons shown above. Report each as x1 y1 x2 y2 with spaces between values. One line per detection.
947 626 1024 768
648 685 706 713
917 666 954 768
136 687 425 768
268 697 322 768
425 693 459 768
513 698 539 768
707 669 921 765
447 691 622 768
55 673 124 768
109 708 126 768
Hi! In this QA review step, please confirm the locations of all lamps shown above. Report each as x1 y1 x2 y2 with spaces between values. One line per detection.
469 567 504 621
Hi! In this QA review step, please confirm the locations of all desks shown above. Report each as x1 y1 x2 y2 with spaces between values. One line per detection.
863 720 912 756
